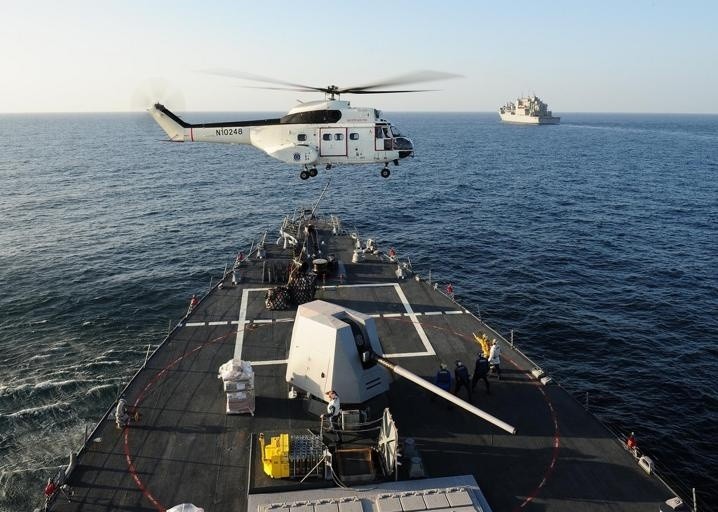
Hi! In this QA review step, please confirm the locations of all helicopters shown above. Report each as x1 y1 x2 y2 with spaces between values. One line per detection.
146 59 467 180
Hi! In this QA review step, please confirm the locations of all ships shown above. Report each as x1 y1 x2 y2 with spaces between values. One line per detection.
501 91 562 124
30 183 716 511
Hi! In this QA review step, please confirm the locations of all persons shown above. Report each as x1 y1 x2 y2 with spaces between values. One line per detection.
187 294 198 312
472 353 492 395
44 477 56 498
435 362 451 393
446 283 453 294
390 247 395 257
232 267 241 285
487 338 502 380
238 252 244 260
472 332 492 359
320 391 343 442
454 360 473 404
